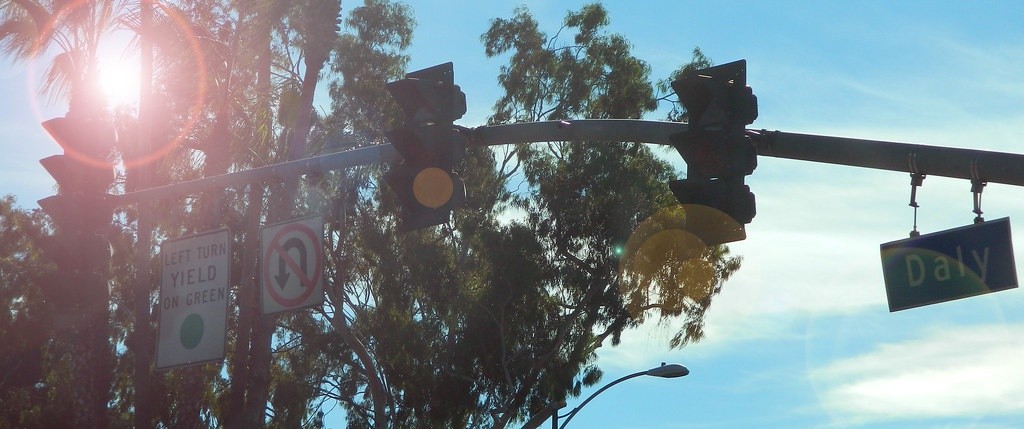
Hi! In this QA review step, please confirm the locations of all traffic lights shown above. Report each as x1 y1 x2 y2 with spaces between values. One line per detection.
670 74 738 226
385 78 451 215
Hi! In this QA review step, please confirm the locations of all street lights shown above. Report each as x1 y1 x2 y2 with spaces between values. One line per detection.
552 362 690 429
